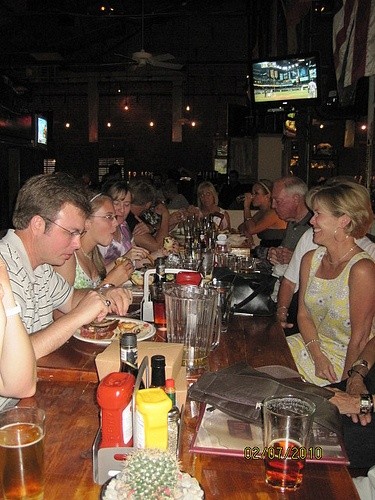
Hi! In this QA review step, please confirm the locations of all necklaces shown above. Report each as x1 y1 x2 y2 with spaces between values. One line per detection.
81 247 92 259
327 244 357 264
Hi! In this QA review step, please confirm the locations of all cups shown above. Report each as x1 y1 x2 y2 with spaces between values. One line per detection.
263 394 316 489
165 285 221 376
174 215 255 282
0 407 50 500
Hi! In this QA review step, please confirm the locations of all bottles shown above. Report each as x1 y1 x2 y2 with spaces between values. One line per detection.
97 332 177 451
150 256 168 332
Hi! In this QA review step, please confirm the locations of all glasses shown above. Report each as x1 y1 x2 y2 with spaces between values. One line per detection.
31 214 87 240
88 215 117 222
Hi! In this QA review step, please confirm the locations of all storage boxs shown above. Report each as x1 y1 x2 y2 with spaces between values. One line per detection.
230 247 250 257
95 341 187 412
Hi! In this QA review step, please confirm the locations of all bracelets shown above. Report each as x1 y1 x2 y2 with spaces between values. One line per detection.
277 306 288 312
100 283 115 295
348 370 365 379
306 339 319 346
369 393 374 413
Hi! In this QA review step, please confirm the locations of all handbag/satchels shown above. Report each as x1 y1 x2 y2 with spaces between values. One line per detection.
221 274 276 316
188 362 344 438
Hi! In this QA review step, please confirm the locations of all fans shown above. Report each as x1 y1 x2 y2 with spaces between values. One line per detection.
114 0 182 70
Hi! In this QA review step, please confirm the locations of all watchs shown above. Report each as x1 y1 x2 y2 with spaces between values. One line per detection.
6 301 21 316
360 393 370 416
353 360 371 370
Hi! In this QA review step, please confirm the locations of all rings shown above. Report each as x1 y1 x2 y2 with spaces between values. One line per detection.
106 300 111 306
269 258 271 261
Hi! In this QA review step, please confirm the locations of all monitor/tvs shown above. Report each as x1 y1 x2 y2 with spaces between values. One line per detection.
250 52 321 109
34 113 48 149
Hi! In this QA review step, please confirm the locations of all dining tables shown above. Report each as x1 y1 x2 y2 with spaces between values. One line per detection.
37 234 300 381
0 382 361 500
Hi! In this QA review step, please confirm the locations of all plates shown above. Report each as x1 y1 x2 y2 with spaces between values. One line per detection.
74 317 156 344
120 279 157 296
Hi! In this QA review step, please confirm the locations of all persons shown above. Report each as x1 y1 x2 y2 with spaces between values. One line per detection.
189 170 252 209
322 335 375 477
188 181 230 229
286 174 375 387
239 175 315 312
307 79 316 98
0 172 189 414
276 228 375 337
